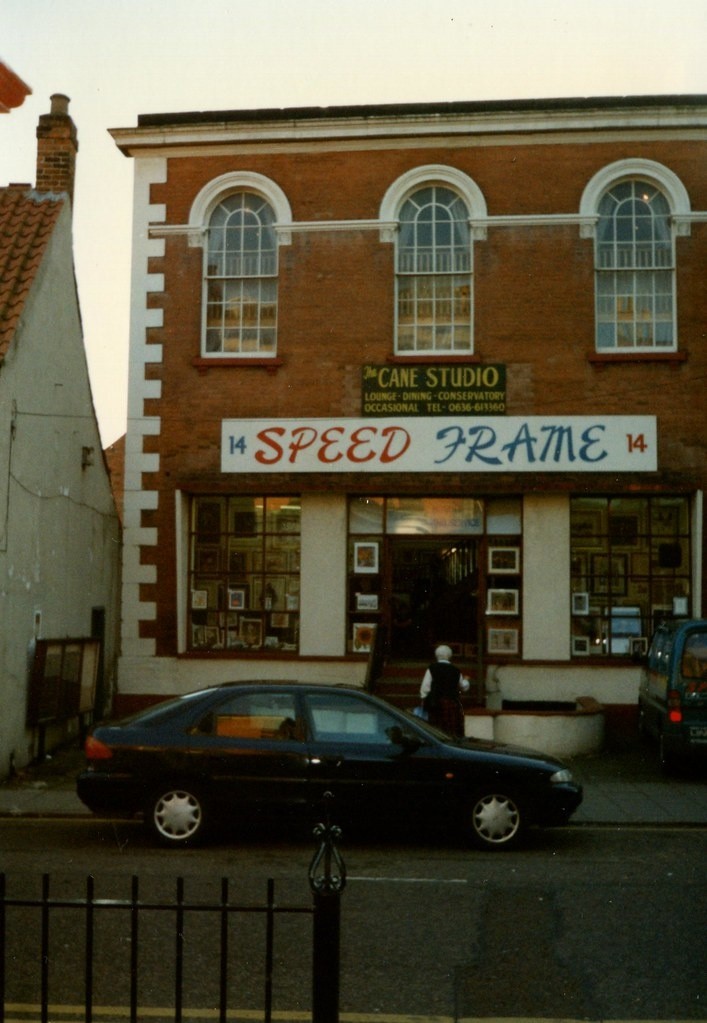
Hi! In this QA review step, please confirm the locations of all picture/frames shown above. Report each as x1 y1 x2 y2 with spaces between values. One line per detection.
485 508 640 653
227 588 244 610
238 618 263 649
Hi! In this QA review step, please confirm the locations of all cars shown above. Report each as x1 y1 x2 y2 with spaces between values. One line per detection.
74 680 586 853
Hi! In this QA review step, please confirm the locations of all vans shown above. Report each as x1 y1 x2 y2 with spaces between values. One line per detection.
631 617 707 767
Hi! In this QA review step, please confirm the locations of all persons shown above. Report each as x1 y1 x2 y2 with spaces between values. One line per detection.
420 646 470 738
